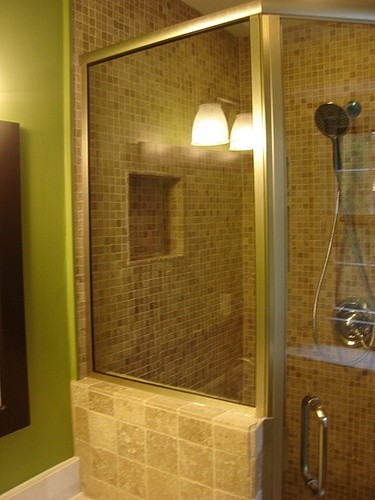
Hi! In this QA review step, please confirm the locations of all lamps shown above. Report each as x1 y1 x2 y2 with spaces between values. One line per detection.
191 86 229 148
229 95 253 150
